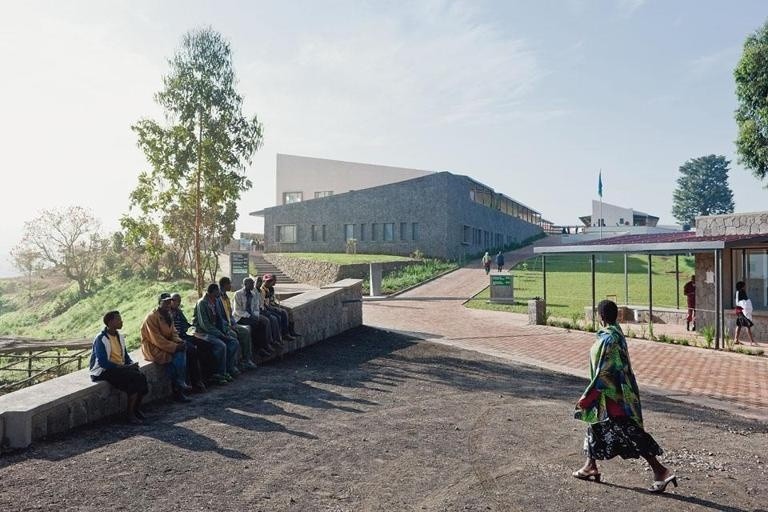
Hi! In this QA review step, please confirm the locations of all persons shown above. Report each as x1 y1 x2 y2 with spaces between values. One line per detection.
732 279 760 347
495 250 504 272
141 292 193 403
193 284 240 384
269 275 302 342
232 278 277 357
480 251 492 275
259 274 286 346
90 309 150 426
251 277 285 351
597 171 604 198
683 275 700 330
217 277 258 377
169 295 213 393
570 299 678 495
248 237 265 252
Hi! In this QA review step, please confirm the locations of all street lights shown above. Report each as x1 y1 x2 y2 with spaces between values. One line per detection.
187 103 205 297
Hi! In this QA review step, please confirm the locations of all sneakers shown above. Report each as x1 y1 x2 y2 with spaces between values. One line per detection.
128 410 147 425
686 326 696 331
173 382 192 402
195 358 258 393
258 331 302 358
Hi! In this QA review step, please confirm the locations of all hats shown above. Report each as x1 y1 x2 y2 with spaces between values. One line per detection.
243 274 277 285
157 292 181 304
219 277 232 288
691 275 695 280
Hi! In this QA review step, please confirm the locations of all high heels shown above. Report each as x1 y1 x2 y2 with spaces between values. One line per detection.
647 474 678 493
734 339 760 346
571 467 600 482
